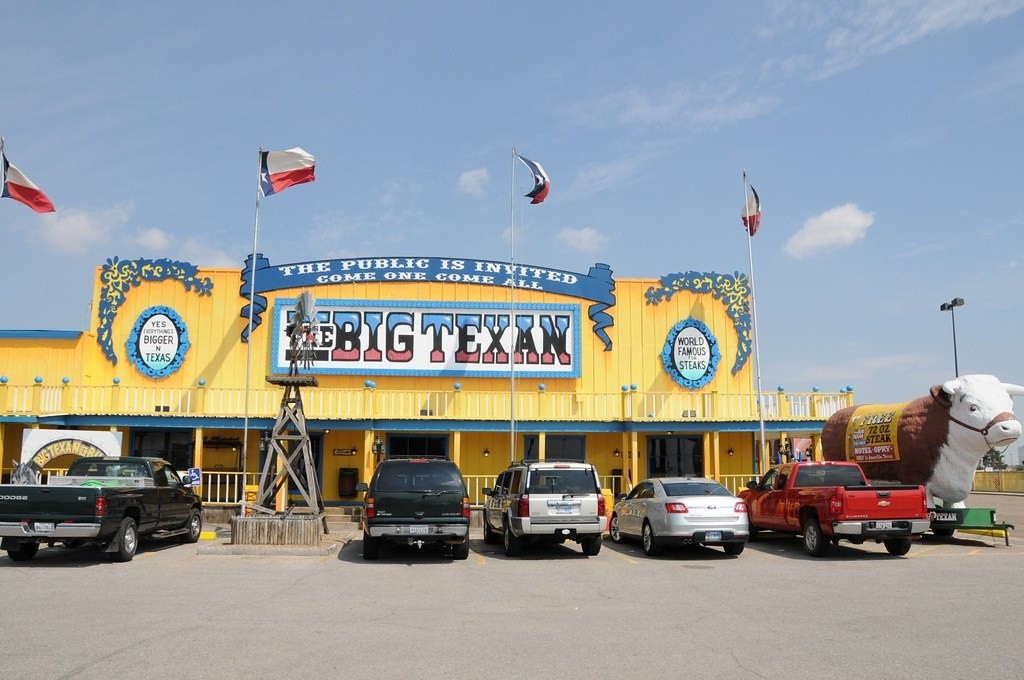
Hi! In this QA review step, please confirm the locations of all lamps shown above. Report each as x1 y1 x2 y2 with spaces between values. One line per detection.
484 448 489 457
351 446 357 455
614 449 619 457
728 448 733 456
231 446 237 452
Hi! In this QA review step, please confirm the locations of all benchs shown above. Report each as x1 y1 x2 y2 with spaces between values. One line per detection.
191 468 243 503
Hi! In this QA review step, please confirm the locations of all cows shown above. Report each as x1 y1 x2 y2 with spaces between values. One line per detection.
821 374 1023 512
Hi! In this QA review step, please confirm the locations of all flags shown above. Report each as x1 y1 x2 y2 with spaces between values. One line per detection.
742 184 762 236
517 155 550 204
260 147 316 197
0 152 57 213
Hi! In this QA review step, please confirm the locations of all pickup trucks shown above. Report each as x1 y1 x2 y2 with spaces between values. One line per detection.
742 460 930 555
0 455 205 563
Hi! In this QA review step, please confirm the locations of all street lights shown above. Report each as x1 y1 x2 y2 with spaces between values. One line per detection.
941 298 966 378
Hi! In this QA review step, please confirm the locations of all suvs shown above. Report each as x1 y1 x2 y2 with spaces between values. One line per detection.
355 458 472 559
484 459 608 554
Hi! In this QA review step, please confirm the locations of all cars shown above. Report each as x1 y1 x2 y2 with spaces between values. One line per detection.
612 476 749 555
